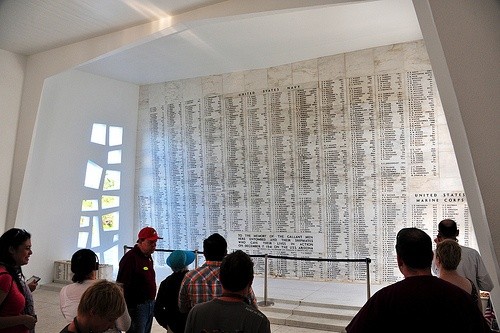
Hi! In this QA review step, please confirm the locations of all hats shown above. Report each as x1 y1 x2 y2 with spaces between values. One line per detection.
165 250 197 268
137 225 164 241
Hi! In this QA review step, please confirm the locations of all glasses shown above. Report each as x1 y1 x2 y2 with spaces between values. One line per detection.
11 228 27 245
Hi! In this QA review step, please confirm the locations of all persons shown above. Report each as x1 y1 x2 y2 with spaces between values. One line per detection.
345 228 491 333
184 250 271 333
178 233 258 315
116 226 163 333
0 228 38 333
431 219 494 296
59 249 131 333
60 279 126 333
435 239 483 312
154 250 196 333
